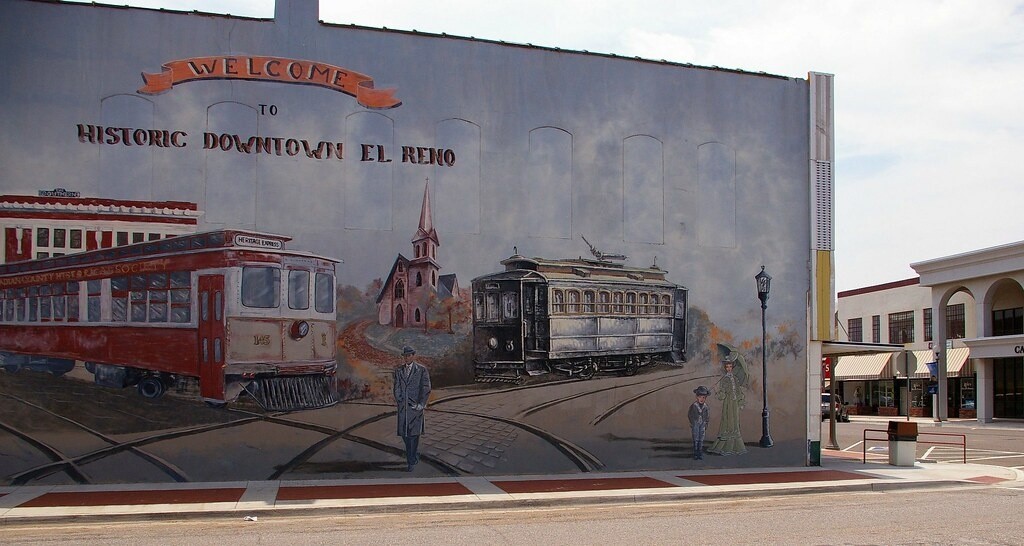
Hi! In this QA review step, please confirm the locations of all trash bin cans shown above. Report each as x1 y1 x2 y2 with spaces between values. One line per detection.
887 420 919 466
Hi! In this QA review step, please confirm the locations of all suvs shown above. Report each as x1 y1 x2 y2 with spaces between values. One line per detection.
821 393 850 423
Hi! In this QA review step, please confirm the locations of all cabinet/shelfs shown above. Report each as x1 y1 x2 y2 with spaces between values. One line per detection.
910 380 924 408
960 378 975 409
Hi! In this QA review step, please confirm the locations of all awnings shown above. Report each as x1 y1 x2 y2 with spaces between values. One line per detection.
834 353 893 381
896 348 971 379
